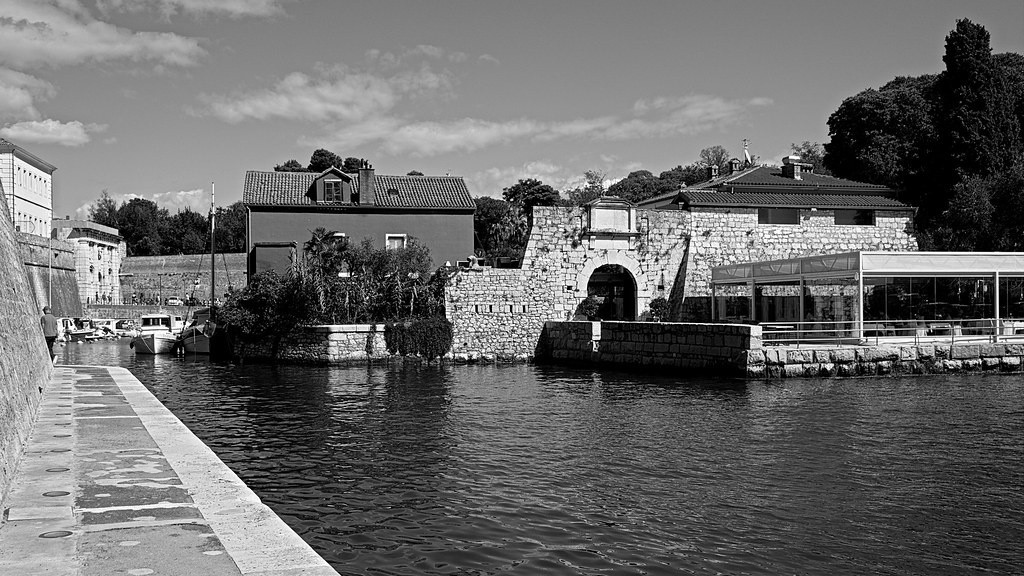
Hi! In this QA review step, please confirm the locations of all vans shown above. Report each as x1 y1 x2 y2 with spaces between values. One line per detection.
166 296 180 306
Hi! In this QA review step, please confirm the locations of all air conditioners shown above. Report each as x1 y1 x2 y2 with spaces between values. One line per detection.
455 261 470 270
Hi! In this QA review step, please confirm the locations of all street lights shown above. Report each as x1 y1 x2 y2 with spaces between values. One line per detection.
157 273 163 306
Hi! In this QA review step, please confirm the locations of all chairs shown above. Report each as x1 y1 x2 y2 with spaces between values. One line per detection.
863 322 1024 337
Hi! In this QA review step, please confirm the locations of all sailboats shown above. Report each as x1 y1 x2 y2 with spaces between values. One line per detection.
177 181 236 355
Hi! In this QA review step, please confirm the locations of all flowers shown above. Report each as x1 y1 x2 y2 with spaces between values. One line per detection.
639 295 674 322
569 295 604 322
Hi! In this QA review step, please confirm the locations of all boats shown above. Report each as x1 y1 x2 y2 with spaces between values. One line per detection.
47 238 139 342
129 312 181 355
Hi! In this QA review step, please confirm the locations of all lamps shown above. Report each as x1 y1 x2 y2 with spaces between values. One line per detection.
563 216 587 247
636 217 655 254
